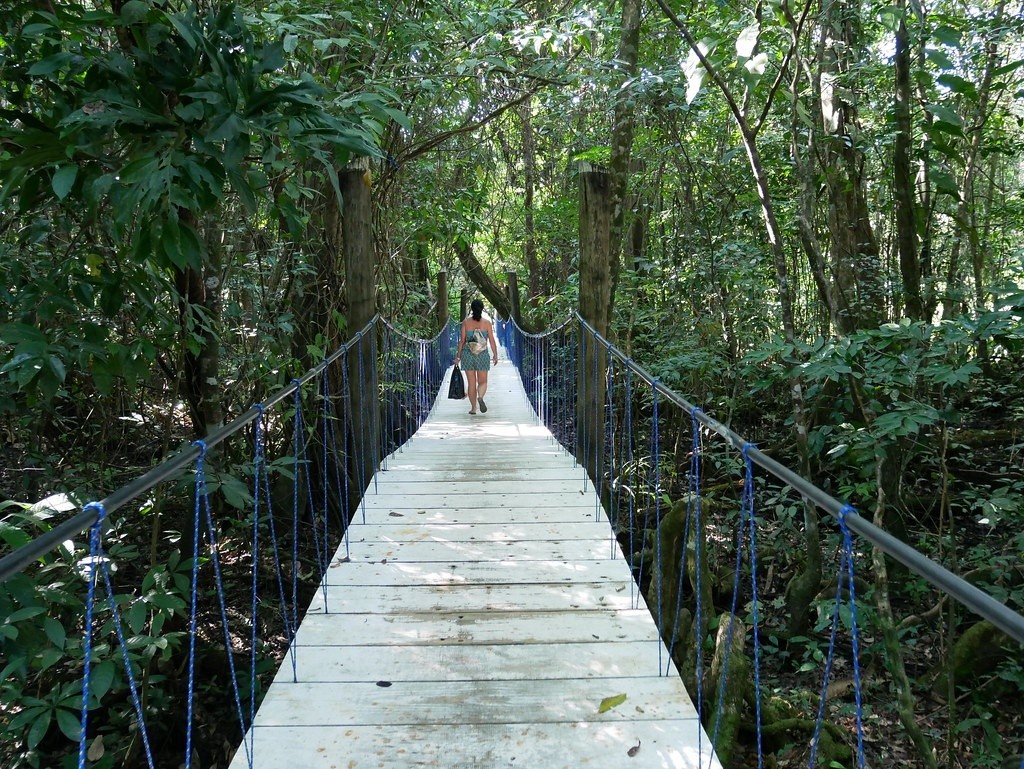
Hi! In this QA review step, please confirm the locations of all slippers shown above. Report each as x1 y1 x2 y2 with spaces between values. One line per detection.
469 410 476 415
478 397 487 413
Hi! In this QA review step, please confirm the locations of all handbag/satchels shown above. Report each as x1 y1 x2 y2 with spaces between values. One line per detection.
448 364 465 399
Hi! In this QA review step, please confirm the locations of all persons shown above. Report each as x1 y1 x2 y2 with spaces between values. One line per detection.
454 299 497 414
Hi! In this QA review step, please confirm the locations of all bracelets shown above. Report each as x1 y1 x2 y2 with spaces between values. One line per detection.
457 357 460 361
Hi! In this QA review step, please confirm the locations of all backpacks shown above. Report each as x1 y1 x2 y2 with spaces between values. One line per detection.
464 317 487 355
